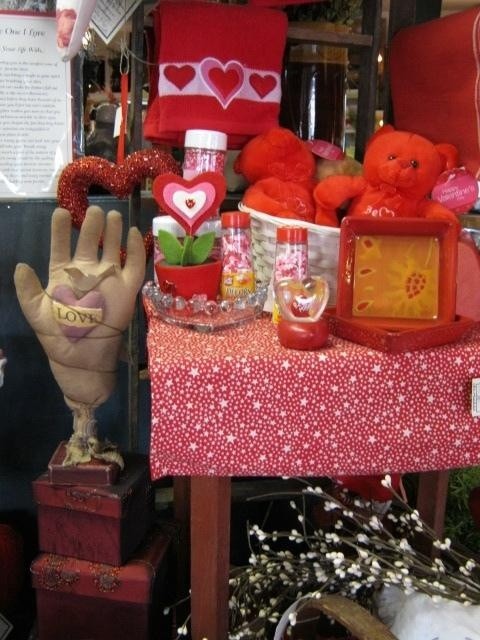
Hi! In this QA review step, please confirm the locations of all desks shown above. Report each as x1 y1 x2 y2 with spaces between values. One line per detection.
146 312 480 639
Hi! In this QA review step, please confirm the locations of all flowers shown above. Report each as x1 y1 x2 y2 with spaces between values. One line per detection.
164 476 479 639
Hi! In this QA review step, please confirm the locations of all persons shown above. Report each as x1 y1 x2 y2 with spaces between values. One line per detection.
13 204 146 470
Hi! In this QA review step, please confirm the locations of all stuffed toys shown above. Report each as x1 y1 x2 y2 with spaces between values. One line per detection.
224 125 461 239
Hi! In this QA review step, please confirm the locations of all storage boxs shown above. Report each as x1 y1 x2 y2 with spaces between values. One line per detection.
30 439 174 640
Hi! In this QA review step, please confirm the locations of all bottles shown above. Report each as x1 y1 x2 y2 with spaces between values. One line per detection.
272 224 309 326
182 130 228 221
219 211 255 301
